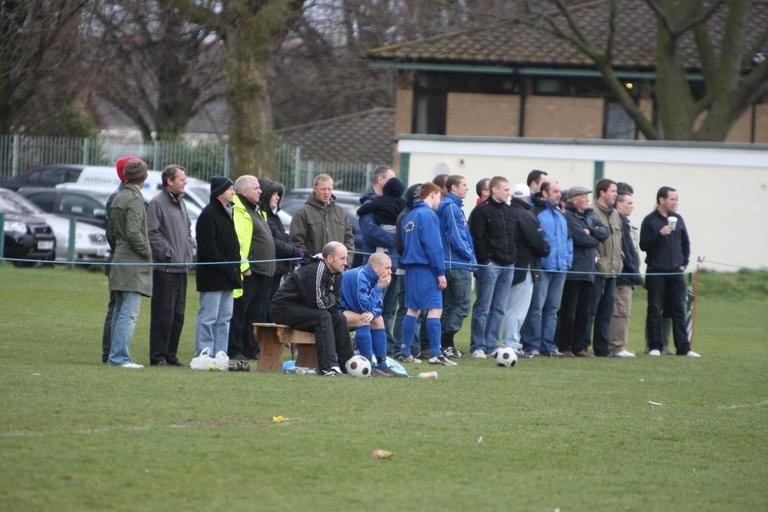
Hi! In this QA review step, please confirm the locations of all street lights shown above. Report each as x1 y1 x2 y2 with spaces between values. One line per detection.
750 52 764 142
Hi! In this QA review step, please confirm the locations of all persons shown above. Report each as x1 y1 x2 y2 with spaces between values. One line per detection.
105 156 354 369
336 252 409 379
357 167 701 365
269 240 373 379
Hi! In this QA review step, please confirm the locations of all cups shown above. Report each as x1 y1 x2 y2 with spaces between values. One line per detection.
666 216 679 232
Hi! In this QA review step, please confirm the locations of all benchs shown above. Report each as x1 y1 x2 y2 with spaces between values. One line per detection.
253 321 319 374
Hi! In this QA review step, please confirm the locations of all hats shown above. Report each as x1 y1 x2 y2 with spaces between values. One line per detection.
124 158 148 184
115 155 135 182
567 185 593 199
210 176 234 198
510 184 531 198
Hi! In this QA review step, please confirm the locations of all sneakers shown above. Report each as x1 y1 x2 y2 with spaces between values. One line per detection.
318 366 408 377
230 351 249 359
120 360 186 368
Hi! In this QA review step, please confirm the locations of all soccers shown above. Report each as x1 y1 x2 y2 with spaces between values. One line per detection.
494 346 517 368
345 355 371 377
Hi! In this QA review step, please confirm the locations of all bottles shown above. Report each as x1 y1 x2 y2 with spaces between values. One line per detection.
414 369 438 380
295 366 317 376
